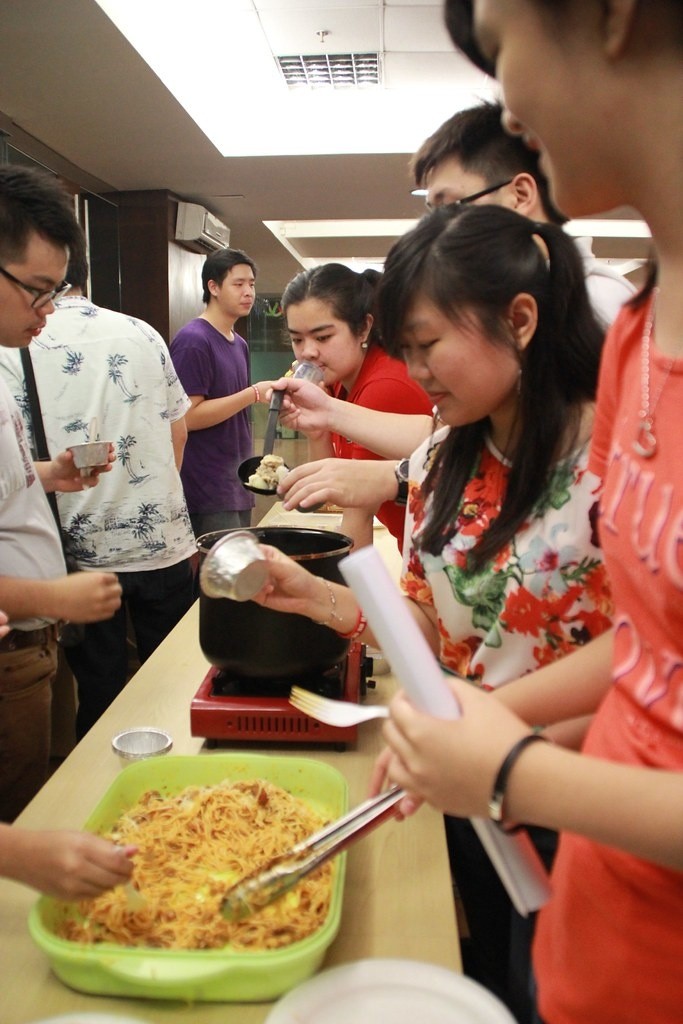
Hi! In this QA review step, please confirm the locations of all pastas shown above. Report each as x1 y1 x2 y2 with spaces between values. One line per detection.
56 778 335 953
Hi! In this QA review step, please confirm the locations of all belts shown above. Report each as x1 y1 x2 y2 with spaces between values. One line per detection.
0 619 63 653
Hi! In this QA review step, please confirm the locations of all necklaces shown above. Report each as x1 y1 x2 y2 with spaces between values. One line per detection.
630 283 683 458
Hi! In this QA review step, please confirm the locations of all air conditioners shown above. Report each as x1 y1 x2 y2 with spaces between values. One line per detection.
175 201 232 251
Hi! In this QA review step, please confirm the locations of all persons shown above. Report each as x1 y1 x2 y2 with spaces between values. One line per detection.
0 160 200 825
0 611 139 903
167 249 280 558
250 103 637 1024
365 0 683 1024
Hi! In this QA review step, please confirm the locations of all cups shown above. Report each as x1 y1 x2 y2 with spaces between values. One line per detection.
292 361 324 389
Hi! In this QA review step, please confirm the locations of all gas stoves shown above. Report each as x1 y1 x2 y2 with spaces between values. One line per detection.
190 643 376 744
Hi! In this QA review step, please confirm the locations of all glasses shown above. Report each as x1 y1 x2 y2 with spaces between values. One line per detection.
0 266 72 308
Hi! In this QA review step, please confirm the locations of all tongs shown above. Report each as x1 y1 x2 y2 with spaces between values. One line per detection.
219 784 406 921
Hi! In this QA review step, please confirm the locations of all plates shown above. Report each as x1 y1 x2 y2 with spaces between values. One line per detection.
264 958 515 1023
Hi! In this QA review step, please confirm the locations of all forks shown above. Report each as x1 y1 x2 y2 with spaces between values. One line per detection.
288 687 387 727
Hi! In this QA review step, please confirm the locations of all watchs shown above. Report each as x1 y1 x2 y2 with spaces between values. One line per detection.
394 458 409 506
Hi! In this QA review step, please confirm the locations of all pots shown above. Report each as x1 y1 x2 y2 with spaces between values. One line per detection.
194 526 356 684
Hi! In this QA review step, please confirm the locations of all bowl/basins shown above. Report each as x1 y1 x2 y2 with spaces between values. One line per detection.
66 440 113 469
277 486 326 512
27 753 349 1003
113 730 173 770
199 529 270 602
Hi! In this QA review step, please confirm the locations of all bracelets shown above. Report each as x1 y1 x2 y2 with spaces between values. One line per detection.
335 602 367 640
251 384 260 403
311 576 344 626
488 734 549 836
